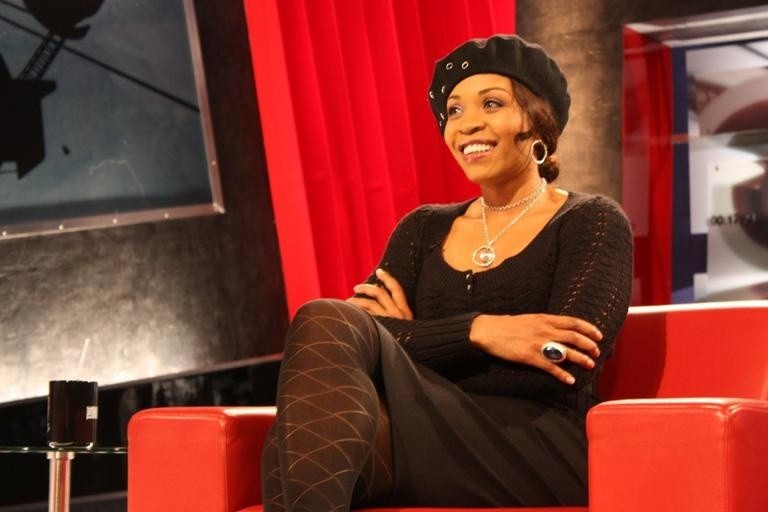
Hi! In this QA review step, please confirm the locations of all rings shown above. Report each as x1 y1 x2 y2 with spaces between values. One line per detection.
541 342 568 363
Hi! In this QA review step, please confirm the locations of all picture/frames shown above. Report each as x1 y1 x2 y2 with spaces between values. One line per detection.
0 0 226 239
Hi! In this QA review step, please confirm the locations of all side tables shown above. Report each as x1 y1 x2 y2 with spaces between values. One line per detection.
0 446 128 512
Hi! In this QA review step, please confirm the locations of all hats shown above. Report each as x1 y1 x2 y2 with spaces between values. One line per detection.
427 33 572 140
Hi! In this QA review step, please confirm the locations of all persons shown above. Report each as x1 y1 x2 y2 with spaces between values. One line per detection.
261 34 633 512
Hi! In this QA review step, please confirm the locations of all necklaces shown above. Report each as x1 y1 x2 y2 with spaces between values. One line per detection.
471 177 547 267
483 187 541 210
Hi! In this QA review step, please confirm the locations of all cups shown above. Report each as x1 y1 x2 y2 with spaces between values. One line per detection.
45 378 98 453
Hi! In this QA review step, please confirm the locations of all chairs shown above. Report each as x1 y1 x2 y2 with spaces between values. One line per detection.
126 300 768 512
